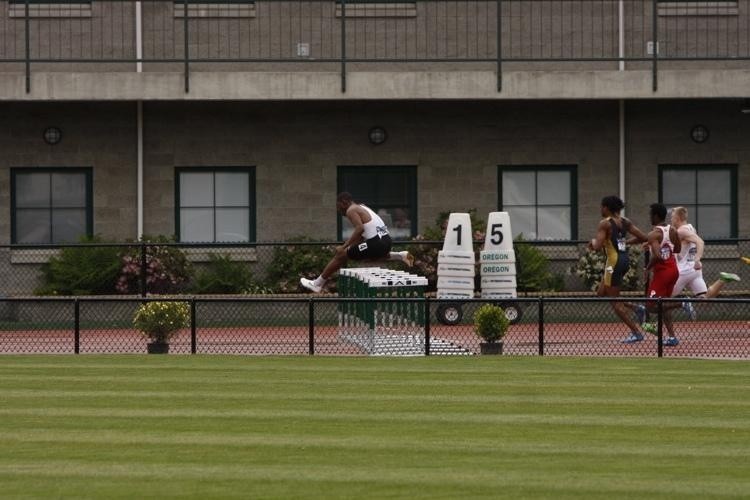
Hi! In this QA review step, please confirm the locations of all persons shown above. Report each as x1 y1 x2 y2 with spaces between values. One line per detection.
587 193 649 343
298 190 414 292
643 204 697 346
640 207 741 336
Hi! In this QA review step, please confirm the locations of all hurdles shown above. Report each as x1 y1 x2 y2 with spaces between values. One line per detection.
337 264 477 356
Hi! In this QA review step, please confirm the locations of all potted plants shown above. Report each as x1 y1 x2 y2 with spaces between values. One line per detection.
473 304 507 354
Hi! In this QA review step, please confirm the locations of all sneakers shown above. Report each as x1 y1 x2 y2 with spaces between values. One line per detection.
662 337 679 346
399 251 414 267
720 272 741 282
682 296 697 321
624 334 642 343
632 304 646 325
300 277 322 293
641 322 658 336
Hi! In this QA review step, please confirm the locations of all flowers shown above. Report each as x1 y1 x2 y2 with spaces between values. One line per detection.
134 300 191 343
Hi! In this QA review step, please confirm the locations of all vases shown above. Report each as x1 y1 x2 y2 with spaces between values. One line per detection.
147 343 169 354
